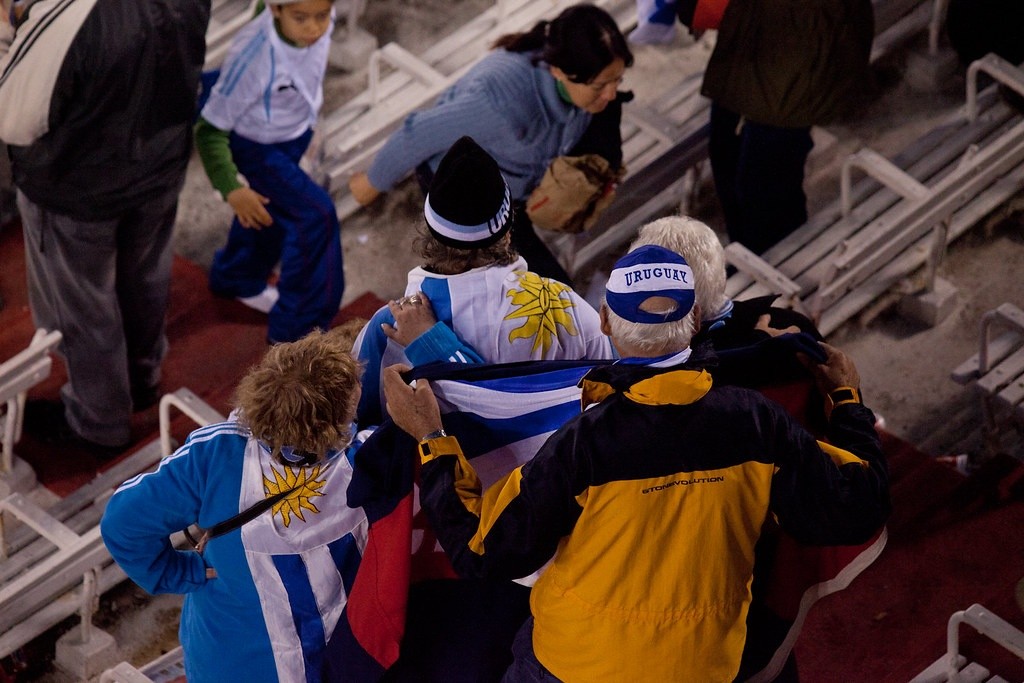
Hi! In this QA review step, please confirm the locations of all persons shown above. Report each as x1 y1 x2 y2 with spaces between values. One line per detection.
702 0 875 280
97 295 489 682
353 4 634 291
381 244 892 683
0 0 211 457
626 214 832 443
196 1 347 346
353 136 616 496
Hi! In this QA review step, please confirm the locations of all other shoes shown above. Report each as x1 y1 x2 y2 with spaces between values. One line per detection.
237 283 280 315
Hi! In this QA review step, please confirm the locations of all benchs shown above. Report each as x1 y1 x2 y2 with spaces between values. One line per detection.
0 1 1022 683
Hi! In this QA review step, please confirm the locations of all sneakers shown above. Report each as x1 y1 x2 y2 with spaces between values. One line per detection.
23 399 126 458
128 382 161 411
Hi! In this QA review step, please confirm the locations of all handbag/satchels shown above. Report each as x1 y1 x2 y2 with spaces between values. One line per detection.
565 85 636 176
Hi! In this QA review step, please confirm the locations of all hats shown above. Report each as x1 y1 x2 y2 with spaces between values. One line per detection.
423 134 513 249
606 244 696 324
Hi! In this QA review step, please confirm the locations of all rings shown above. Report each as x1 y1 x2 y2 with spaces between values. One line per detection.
195 544 200 550
409 295 422 305
399 299 411 308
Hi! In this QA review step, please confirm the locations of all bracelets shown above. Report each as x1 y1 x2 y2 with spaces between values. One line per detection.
423 430 447 441
